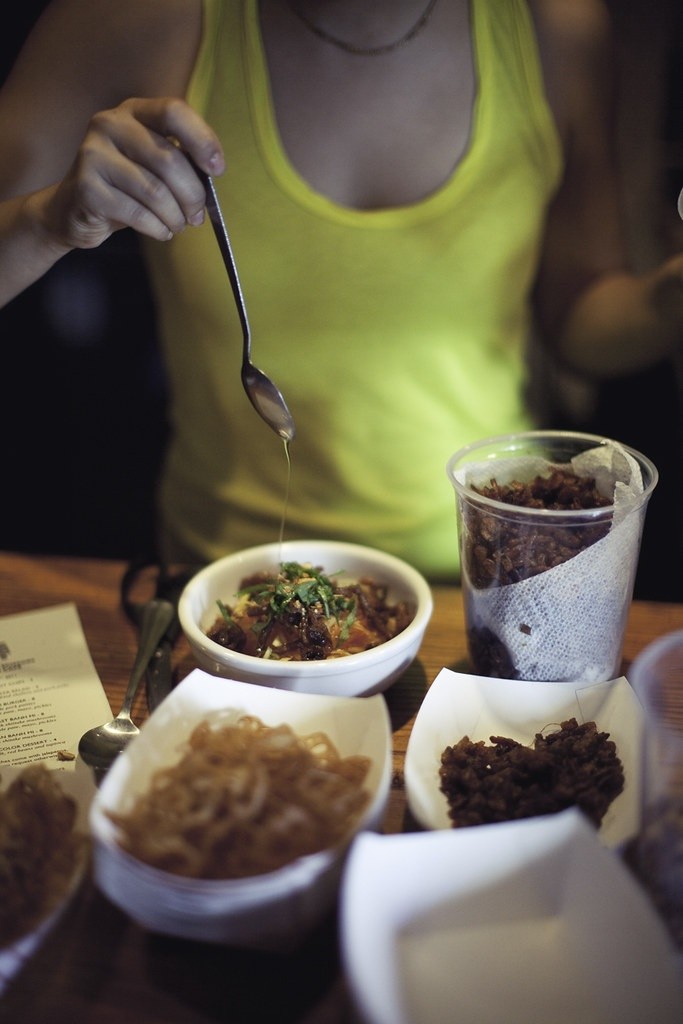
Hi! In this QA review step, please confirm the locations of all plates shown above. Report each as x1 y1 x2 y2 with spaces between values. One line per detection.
339 806 683 1024
0 766 97 991
88 668 393 941
405 666 656 852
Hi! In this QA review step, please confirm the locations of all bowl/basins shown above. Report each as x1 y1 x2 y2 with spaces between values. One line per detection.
177 540 434 698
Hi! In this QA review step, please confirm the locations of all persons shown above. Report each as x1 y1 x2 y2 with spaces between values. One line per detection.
0 0 683 588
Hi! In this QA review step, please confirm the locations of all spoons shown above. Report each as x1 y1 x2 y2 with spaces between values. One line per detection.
79 599 175 768
187 153 297 443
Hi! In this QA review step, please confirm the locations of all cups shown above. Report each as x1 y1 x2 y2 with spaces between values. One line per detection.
446 431 660 683
628 629 683 899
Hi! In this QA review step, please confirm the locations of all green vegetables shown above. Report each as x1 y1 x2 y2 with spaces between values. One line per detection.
234 565 357 643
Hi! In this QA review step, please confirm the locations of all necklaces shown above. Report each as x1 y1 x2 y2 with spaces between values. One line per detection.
286 0 436 56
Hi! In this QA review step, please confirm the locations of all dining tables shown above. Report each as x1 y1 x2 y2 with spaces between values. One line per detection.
0 556 683 1024
